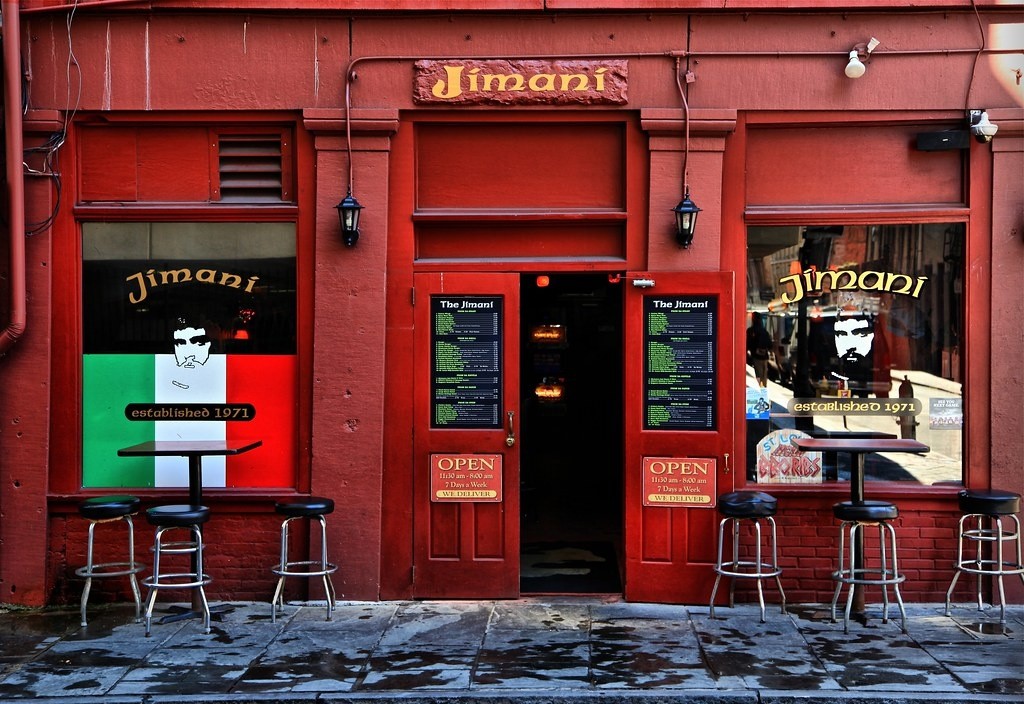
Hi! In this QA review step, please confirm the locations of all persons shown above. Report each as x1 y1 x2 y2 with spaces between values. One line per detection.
747 311 776 389
830 315 875 374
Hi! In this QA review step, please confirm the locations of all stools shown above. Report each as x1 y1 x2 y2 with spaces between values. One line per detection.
709 490 791 623
75 496 146 626
268 495 338 624
945 489 1024 625
140 505 212 638
831 500 910 635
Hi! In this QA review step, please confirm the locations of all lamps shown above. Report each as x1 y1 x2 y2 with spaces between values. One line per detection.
845 50 865 79
333 183 365 248
671 184 703 248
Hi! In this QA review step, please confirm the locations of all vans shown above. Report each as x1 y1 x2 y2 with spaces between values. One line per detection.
745 295 895 401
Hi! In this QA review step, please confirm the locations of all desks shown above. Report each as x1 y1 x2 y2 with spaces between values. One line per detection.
117 440 263 624
790 438 929 629
